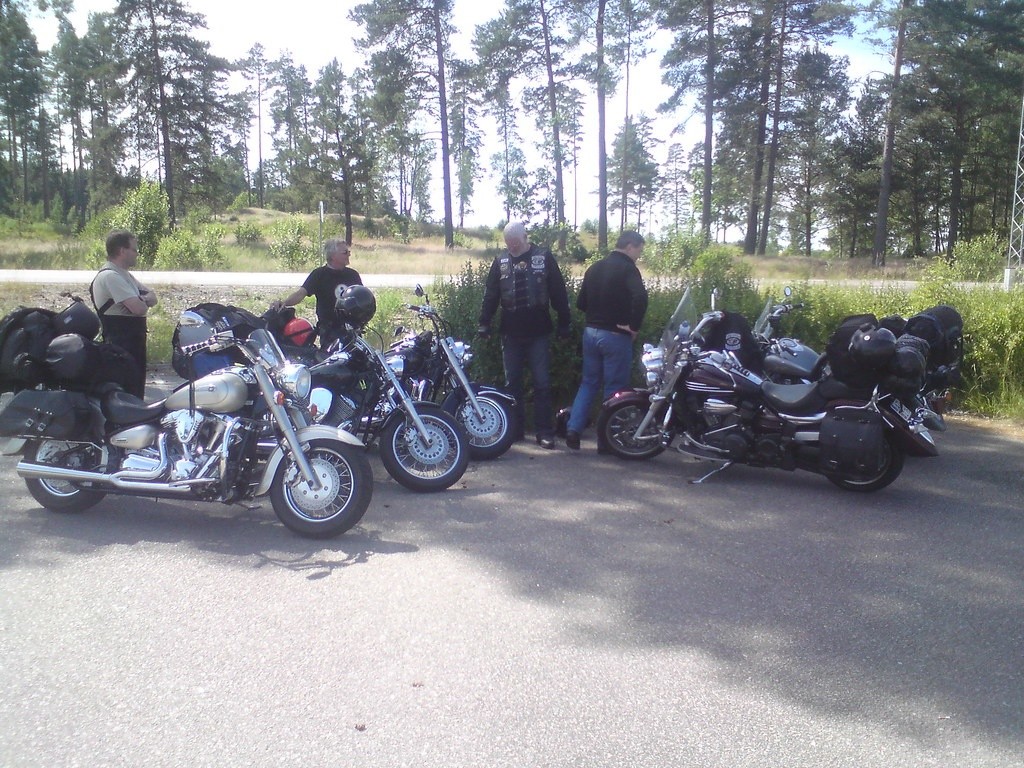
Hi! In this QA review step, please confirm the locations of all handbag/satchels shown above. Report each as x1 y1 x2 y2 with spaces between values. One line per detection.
888 333 931 379
172 303 266 382
900 305 963 352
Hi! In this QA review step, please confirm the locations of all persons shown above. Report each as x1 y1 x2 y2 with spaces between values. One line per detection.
90 229 158 402
565 230 648 450
478 221 572 449
269 240 364 351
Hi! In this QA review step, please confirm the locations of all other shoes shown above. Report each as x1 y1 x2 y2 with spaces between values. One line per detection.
598 447 614 455
537 434 555 449
566 431 580 450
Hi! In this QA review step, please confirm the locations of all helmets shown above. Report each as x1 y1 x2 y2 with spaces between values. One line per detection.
333 284 376 329
50 300 100 342
284 318 314 347
847 323 897 366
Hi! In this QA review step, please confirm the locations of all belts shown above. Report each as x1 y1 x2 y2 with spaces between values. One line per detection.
586 323 631 336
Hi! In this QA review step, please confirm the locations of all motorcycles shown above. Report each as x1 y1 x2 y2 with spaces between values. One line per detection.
0 303 375 540
739 284 964 458
171 279 471 493
345 284 520 462
596 285 939 493
0 290 106 397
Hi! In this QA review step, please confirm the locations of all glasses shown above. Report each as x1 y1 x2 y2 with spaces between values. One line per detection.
504 238 522 249
339 250 350 254
